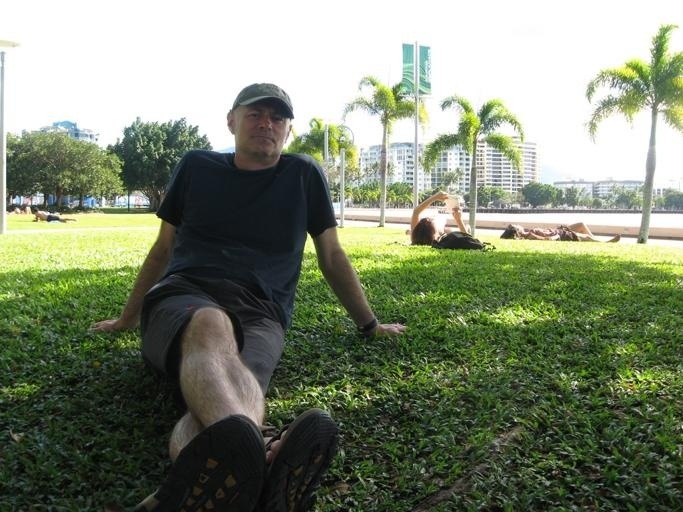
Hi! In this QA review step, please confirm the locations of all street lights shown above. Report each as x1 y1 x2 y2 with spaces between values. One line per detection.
338 136 347 228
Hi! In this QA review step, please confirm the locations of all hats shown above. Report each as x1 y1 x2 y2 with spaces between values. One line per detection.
233 83 294 119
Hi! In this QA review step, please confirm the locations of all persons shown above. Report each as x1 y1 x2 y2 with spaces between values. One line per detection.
406 190 471 245
33 207 76 223
91 83 412 512
500 222 620 242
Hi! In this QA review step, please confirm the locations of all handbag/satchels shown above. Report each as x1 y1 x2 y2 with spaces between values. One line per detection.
439 232 483 249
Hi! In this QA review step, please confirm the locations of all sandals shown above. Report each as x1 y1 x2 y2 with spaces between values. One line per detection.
131 415 266 510
265 408 337 512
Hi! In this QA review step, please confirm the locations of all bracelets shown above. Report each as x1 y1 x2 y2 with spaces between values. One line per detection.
357 318 378 332
